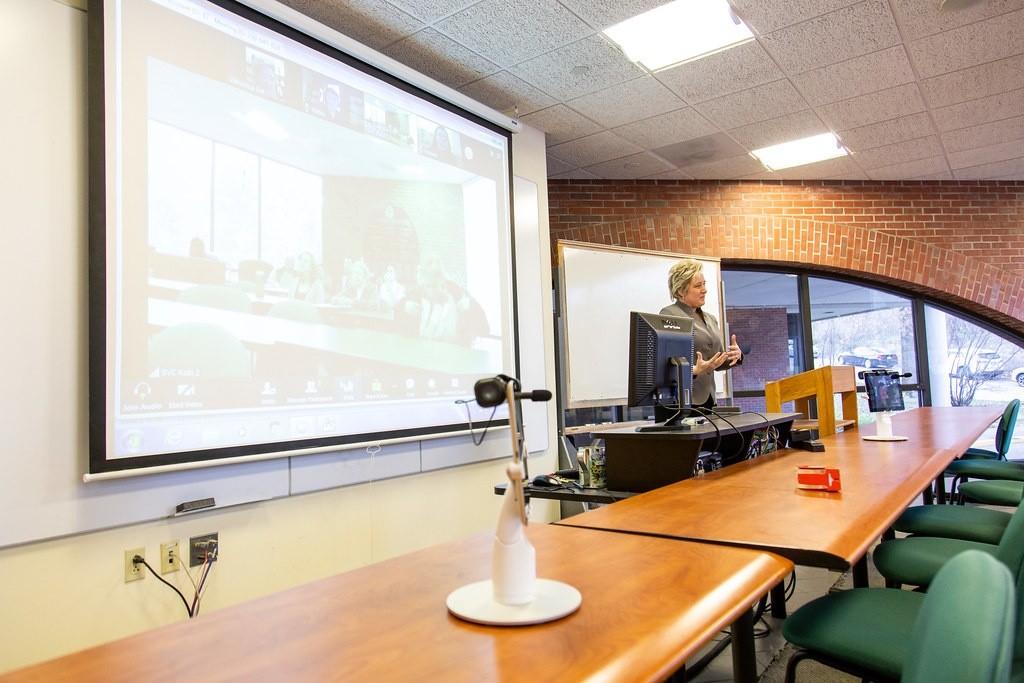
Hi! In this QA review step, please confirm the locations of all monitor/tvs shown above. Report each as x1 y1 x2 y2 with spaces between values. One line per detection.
627 310 695 432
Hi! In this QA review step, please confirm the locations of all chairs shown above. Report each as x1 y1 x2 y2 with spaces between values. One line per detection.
782 399 1024 683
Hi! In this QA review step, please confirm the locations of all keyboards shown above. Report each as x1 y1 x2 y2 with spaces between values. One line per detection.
554 467 580 480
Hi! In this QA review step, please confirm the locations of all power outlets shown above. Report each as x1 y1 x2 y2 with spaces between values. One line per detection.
189 531 219 568
125 546 146 583
160 538 181 574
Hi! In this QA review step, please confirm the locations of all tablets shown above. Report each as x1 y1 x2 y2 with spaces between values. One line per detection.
863 371 905 412
507 379 530 527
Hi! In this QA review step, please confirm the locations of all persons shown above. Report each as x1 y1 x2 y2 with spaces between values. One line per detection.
876 383 887 407
659 258 745 417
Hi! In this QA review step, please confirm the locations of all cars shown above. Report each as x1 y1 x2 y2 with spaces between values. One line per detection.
788 340 819 358
838 346 898 369
1011 367 1024 387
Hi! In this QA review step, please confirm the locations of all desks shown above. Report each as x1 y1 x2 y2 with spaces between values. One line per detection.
0 521 795 683
493 480 639 505
847 406 1007 505
549 432 958 683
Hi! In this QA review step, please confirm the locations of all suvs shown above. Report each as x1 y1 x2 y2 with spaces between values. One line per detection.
948 349 1005 380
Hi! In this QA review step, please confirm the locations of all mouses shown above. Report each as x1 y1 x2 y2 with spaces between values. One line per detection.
533 476 562 486
